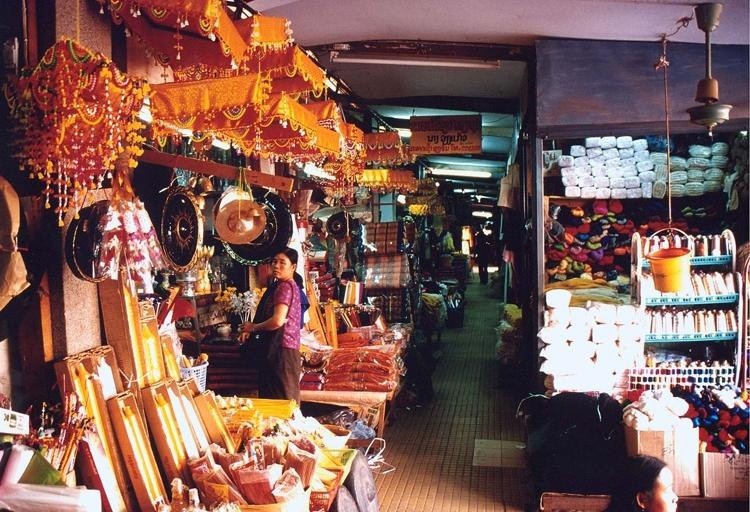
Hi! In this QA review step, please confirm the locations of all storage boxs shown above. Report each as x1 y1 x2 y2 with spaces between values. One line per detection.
701 450 749 500
624 421 701 498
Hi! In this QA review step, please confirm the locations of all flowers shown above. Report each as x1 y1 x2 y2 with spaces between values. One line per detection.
230 289 259 323
214 284 235 313
251 287 266 304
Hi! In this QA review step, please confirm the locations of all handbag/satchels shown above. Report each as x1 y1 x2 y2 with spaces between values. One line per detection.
239 326 284 373
521 390 626 495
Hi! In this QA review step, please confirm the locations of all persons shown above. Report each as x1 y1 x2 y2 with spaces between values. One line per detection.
600 454 679 512
238 246 301 408
473 232 491 284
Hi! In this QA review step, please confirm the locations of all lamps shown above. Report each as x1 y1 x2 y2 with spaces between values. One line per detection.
329 48 503 70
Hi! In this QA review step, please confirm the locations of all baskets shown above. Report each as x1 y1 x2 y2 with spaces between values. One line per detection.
179 359 209 392
628 366 734 391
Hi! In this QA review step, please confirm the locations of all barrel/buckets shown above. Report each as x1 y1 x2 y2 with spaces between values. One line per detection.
643 227 695 294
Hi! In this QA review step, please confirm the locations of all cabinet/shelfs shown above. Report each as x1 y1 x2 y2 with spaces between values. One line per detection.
532 118 749 393
195 337 258 396
297 389 396 456
631 228 745 386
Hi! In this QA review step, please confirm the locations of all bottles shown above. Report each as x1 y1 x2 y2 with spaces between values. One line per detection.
171 478 188 512
153 496 170 512
184 487 206 512
